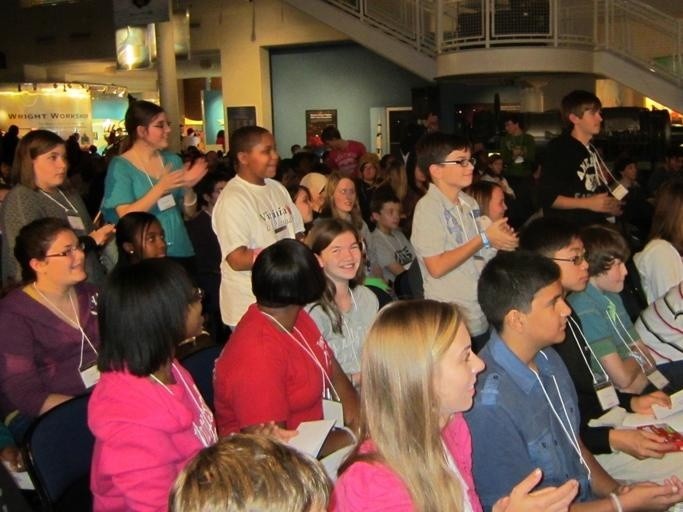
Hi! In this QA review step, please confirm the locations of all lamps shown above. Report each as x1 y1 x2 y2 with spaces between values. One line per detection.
18 80 126 97
114 0 191 71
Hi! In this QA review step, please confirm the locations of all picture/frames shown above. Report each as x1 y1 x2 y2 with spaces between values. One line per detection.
305 109 337 149
227 106 256 150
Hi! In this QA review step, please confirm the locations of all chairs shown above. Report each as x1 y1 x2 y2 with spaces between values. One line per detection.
364 284 392 310
394 270 414 300
21 341 223 512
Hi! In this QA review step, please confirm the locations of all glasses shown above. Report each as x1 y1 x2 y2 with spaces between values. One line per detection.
553 252 591 266
439 157 476 167
187 286 203 303
45 242 86 257
154 120 172 129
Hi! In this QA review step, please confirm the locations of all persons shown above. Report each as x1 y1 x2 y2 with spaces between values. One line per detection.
0 90 682 511
